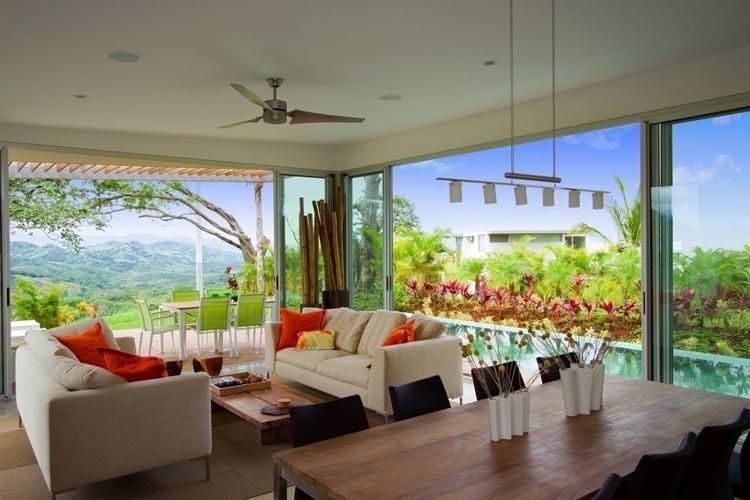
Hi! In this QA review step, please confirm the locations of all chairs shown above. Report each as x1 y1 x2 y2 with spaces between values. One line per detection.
135 288 275 363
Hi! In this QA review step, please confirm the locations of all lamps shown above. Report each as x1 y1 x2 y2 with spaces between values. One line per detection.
435 1 611 211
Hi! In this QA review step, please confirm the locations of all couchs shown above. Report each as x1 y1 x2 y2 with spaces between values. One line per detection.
14 318 213 499
267 307 461 414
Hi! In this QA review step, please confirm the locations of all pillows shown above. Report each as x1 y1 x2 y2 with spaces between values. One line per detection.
366 319 415 369
274 308 326 353
296 329 335 351
97 346 169 382
53 321 111 370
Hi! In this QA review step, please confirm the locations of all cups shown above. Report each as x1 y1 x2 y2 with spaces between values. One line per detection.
278 398 291 409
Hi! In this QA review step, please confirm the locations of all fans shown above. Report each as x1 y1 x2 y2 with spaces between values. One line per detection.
216 76 363 136
